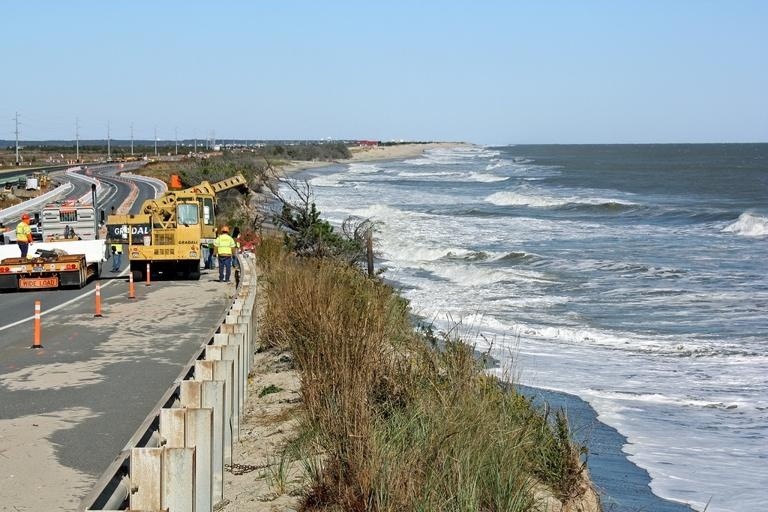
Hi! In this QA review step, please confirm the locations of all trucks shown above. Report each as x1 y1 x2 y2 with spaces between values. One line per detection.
0 196 108 294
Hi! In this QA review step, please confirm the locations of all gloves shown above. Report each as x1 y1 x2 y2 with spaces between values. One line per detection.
121 251 123 255
30 242 33 246
114 251 118 256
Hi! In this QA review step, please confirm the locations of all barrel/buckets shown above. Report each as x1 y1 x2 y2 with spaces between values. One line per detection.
143 236 151 246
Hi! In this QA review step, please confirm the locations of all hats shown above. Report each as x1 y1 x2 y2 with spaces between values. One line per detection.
22 214 30 219
221 226 229 233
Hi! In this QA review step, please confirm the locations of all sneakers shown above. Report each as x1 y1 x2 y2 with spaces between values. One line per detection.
219 277 230 282
113 270 121 274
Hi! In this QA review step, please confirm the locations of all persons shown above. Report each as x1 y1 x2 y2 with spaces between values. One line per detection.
201 224 243 283
15 213 34 257
110 242 124 273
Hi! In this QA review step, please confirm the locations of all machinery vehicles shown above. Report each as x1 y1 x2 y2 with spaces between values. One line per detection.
105 167 251 282
